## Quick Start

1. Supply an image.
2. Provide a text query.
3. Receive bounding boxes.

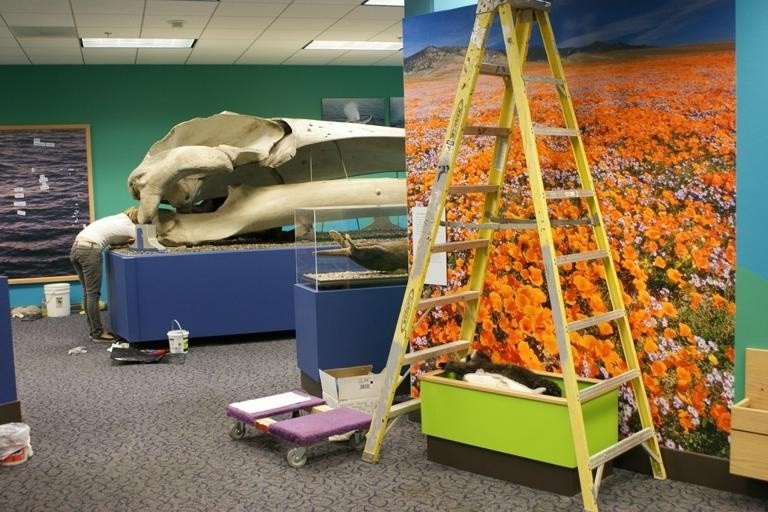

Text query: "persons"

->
[71,206,140,344]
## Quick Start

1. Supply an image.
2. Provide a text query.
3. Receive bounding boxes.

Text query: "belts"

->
[74,240,101,249]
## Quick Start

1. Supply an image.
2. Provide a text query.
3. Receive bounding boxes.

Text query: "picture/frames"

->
[389,96,404,127]
[320,97,386,127]
[0,124,95,285]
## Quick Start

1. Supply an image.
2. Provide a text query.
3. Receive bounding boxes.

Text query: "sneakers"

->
[93,333,120,342]
[89,333,93,339]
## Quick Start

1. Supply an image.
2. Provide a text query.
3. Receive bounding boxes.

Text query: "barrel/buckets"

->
[44,283,71,318]
[167,318,190,354]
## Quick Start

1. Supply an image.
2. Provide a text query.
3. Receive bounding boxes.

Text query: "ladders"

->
[361,1,667,512]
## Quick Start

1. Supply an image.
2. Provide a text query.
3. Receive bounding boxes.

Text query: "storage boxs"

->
[417,363,617,497]
[318,365,404,417]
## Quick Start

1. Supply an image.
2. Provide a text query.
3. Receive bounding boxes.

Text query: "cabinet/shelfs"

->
[291,204,411,399]
[105,229,407,343]
